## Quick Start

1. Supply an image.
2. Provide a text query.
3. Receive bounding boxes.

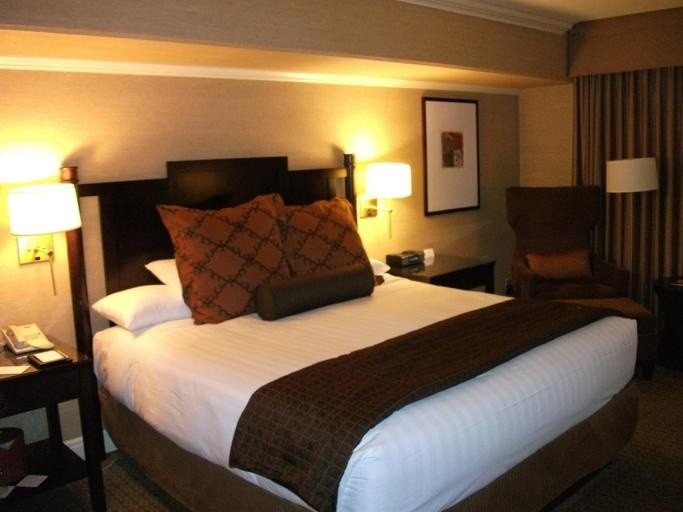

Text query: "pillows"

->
[275,196,375,281]
[253,262,374,320]
[142,258,182,290]
[367,255,392,278]
[155,192,292,325]
[524,249,594,282]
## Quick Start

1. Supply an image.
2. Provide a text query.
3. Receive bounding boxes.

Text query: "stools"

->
[542,295,656,381]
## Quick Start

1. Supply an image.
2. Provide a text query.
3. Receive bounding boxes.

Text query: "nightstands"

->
[0,333,108,511]
[386,252,497,294]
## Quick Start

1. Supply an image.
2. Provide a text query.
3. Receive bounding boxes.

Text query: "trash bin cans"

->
[0,427,30,485]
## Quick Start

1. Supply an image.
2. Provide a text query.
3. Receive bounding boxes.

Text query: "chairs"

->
[505,185,629,300]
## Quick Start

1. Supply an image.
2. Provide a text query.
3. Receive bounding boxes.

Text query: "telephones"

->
[1,323,54,355]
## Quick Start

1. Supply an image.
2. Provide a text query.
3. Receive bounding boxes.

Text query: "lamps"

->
[6,182,83,296]
[87,284,194,332]
[358,161,412,240]
[605,156,660,194]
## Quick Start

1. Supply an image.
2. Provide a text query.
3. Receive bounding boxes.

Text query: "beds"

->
[60,154,640,512]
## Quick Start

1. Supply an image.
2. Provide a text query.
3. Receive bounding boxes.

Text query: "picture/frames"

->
[421,96,481,216]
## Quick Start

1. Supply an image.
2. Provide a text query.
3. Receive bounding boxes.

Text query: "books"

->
[28,349,73,369]
